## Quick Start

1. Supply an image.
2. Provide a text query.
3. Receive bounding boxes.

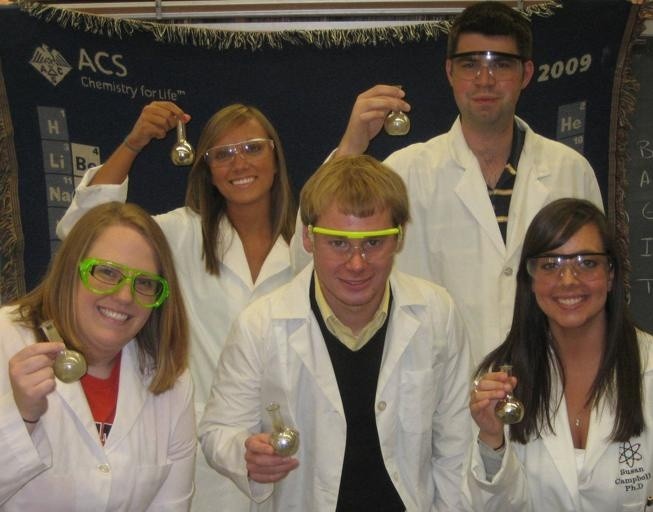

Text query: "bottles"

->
[171,117,195,167]
[38,319,86,385]
[383,84,411,136]
[265,403,299,455]
[494,363,526,426]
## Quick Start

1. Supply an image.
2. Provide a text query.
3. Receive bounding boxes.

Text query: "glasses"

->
[451,50,526,80]
[79,257,171,309]
[308,224,404,257]
[205,138,275,167]
[527,251,617,284]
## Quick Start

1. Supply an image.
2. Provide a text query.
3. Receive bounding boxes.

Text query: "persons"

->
[55,103,290,422]
[466,197,653,511]
[197,154,476,512]
[0,200,197,512]
[290,6,607,378]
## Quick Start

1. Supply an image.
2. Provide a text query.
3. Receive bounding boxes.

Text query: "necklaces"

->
[547,328,603,431]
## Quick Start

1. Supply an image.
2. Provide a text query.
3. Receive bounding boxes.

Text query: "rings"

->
[472,377,482,391]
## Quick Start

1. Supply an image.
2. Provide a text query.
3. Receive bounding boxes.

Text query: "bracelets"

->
[22,414,42,426]
[122,135,145,155]
